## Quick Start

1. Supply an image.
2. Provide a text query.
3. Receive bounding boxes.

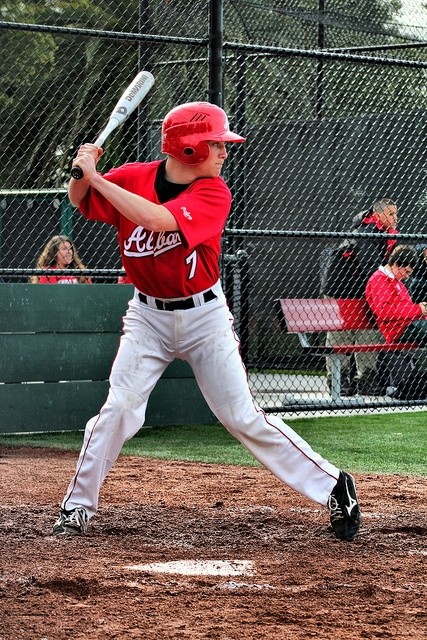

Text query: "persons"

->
[32,236,93,284]
[318,198,399,396]
[365,245,427,347]
[52,100,360,542]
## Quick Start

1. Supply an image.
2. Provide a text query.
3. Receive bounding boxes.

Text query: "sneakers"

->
[326,369,355,396]
[328,471,360,540]
[356,368,380,396]
[49,507,88,538]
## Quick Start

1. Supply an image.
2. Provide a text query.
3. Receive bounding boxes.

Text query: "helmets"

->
[161,101,246,166]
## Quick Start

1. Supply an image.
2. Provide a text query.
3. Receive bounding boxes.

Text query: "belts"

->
[137,289,217,311]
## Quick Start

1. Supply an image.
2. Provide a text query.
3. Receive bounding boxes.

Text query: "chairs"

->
[275,297,427,407]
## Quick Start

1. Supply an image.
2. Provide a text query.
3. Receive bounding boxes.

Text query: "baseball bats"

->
[70,70,155,180]
